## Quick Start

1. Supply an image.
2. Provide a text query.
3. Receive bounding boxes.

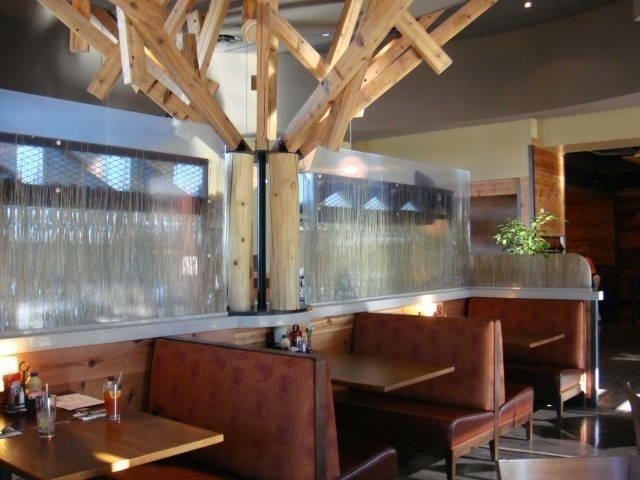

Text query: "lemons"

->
[109,390,122,399]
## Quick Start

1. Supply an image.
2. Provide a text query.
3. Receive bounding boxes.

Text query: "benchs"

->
[469,298,589,420]
[314,356,398,479]
[498,384,534,451]
[350,313,499,479]
[117,339,326,480]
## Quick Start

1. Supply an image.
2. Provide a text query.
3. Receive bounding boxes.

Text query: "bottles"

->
[107,376,120,392]
[2,373,27,403]
[433,303,449,318]
[17,359,30,384]
[9,380,27,417]
[417,311,423,316]
[271,325,314,353]
[299,266,309,310]
[24,370,44,397]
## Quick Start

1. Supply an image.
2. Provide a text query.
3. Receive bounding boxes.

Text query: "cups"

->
[103,382,123,422]
[32,394,57,439]
[37,433,58,447]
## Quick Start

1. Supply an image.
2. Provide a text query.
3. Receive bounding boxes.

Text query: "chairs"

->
[624,380,639,451]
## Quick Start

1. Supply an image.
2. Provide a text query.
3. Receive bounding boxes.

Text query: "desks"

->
[296,350,455,479]
[0,411,226,479]
[494,454,640,480]
[499,333,568,421]
